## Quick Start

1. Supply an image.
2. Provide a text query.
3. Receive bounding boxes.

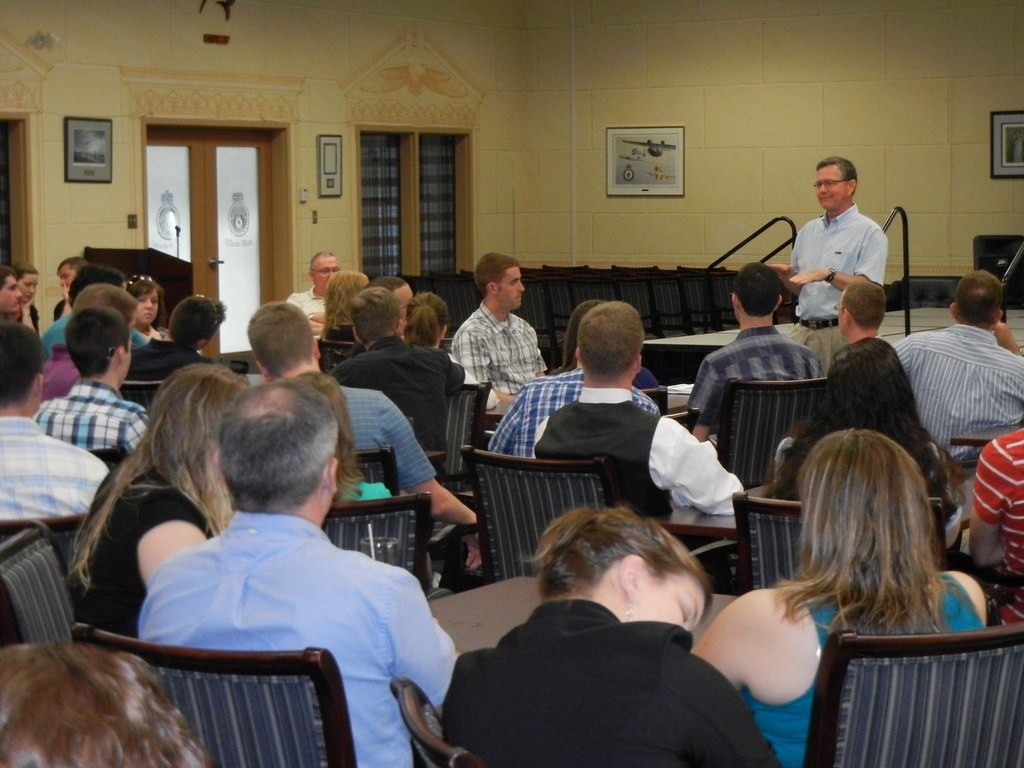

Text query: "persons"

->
[488,299,661,459]
[247,301,482,571]
[690,429,986,768]
[286,251,341,336]
[451,253,549,401]
[328,286,465,452]
[0,260,40,332]
[532,301,744,519]
[33,305,149,454]
[767,157,888,378]
[78,366,251,639]
[319,270,413,343]
[0,318,110,521]
[439,507,783,768]
[766,337,958,518]
[125,295,225,382]
[834,281,886,345]
[40,256,165,401]
[0,642,210,768]
[966,428,1024,626]
[404,291,497,409]
[139,380,456,768]
[890,269,1024,461]
[686,261,823,443]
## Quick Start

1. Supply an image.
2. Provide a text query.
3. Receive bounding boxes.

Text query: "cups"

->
[360,537,399,569]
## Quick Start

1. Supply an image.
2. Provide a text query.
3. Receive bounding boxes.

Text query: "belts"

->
[798,318,838,330]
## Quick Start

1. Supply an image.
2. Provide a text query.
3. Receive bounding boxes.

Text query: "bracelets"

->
[825,268,835,283]
[309,313,313,319]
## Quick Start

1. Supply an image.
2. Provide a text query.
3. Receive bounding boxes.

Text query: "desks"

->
[641,306,1024,394]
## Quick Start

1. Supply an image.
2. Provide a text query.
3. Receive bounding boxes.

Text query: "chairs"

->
[0,233,1024,768]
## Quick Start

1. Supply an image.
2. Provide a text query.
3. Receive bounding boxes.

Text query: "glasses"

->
[126,273,154,292]
[813,179,847,189]
[309,266,341,274]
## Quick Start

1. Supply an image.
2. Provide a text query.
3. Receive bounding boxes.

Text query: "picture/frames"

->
[315,134,342,198]
[64,117,113,183]
[989,110,1024,178]
[606,126,685,198]
